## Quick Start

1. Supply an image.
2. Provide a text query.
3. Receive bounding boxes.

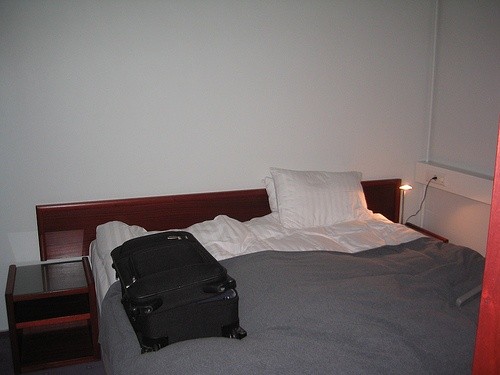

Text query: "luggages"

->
[111,231,248,353]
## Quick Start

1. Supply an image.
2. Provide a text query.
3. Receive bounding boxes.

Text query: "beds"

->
[36,178,486,375]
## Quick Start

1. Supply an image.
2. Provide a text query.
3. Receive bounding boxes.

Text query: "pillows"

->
[263,166,371,230]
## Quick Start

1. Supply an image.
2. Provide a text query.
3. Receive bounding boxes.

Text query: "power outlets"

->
[424,171,449,188]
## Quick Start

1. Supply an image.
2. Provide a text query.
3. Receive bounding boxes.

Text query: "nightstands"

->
[4,257,99,375]
